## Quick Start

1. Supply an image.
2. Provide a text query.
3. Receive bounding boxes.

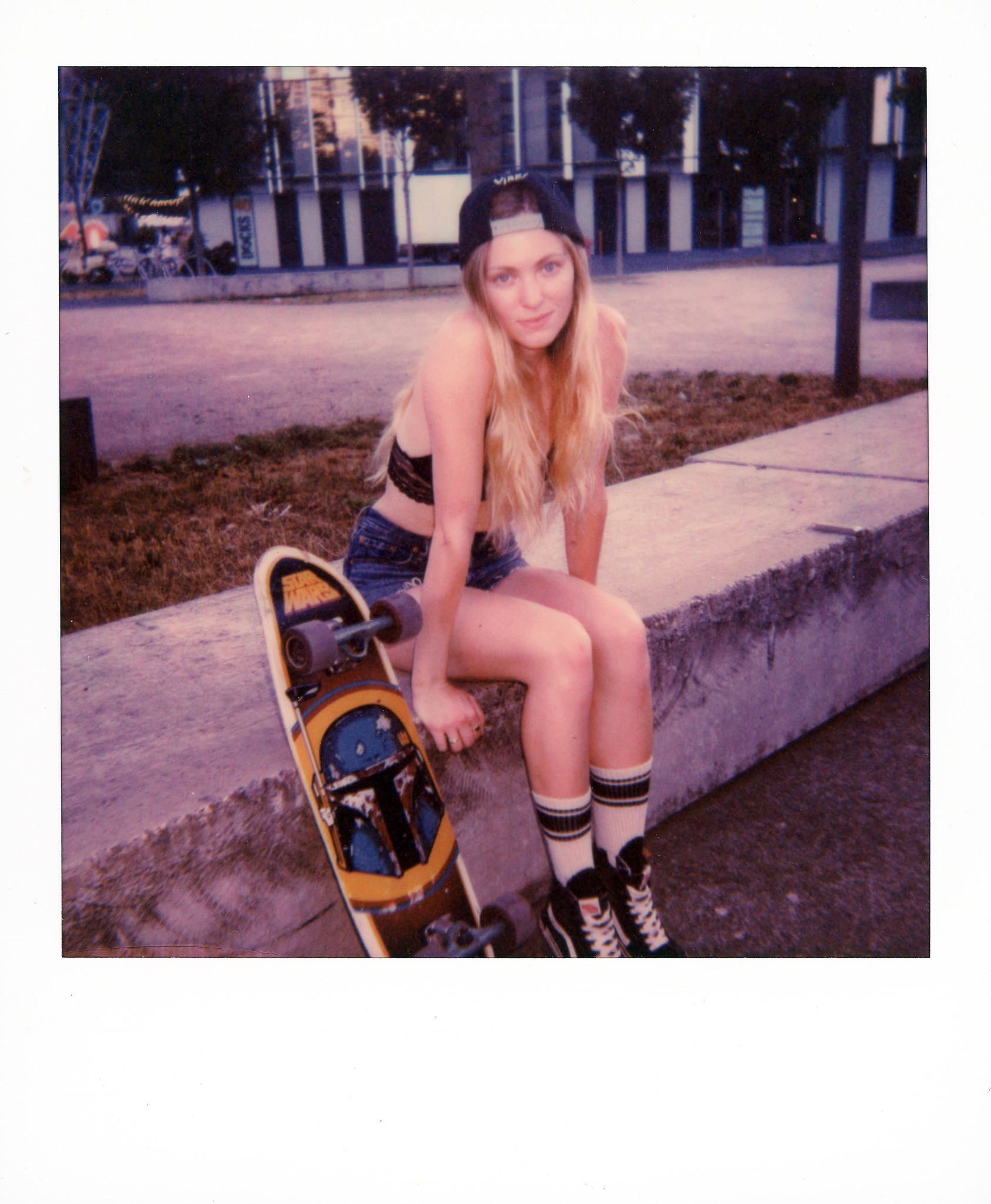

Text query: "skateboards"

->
[251,544,537,957]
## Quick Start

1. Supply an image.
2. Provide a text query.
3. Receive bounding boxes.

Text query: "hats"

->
[455,166,587,272]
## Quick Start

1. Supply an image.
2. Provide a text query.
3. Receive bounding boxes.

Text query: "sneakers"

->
[597,864,688,958]
[538,886,637,959]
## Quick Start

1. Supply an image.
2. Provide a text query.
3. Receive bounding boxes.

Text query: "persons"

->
[342,169,692,958]
[135,223,190,260]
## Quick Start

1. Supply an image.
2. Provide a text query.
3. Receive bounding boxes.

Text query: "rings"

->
[449,737,461,744]
[472,724,481,732]
